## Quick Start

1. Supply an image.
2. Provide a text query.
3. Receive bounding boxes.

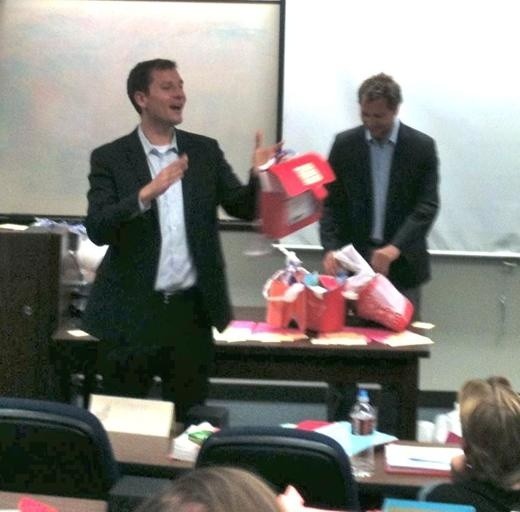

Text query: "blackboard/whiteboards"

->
[0,0,285,233]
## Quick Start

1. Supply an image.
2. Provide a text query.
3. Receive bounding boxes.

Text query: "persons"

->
[136,462,305,512]
[419,375,520,511]
[77,58,286,425]
[316,70,442,440]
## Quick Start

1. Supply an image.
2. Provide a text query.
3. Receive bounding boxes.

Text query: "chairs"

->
[0,395,520,512]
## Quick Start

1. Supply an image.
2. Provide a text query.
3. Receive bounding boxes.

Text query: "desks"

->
[48,303,431,441]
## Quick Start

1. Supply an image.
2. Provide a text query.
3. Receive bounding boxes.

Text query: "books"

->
[169,419,222,462]
[385,443,468,477]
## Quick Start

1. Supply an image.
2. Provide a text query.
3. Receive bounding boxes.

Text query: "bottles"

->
[350,388,376,479]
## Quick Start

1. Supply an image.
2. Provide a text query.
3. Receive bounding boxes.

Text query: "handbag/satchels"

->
[262,266,415,333]
[258,148,337,236]
